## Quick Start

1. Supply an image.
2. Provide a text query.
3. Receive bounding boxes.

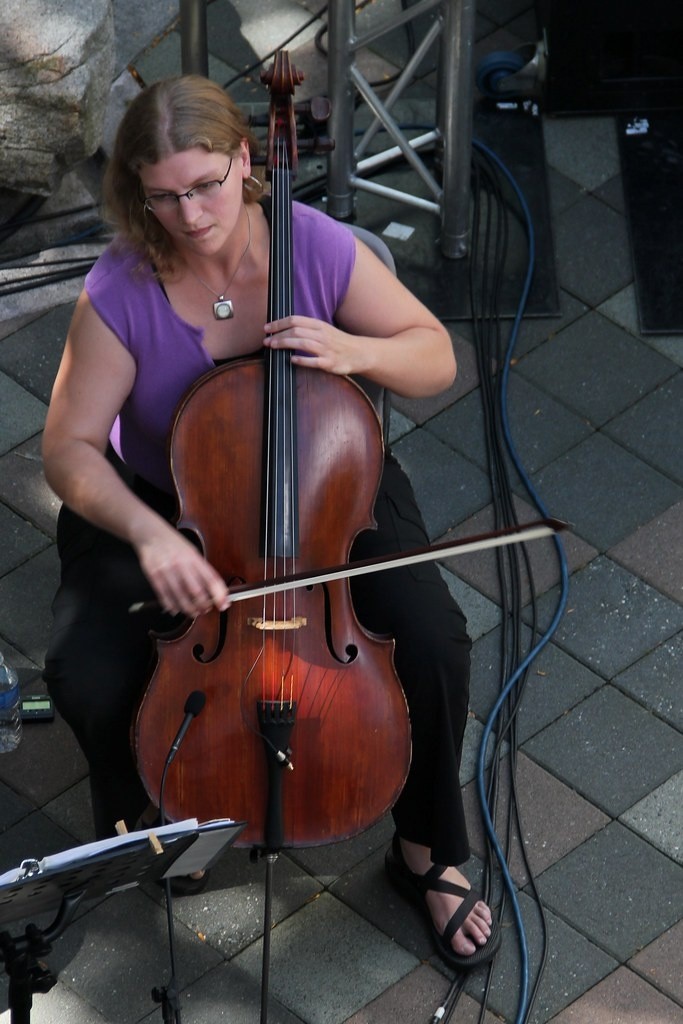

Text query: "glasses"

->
[143,158,233,210]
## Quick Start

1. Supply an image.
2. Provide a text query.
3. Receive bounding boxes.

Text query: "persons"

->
[42,73,503,966]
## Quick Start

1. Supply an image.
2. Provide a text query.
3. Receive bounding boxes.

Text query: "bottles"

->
[0,652,23,754]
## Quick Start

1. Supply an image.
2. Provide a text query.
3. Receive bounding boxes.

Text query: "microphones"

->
[167,692,206,768]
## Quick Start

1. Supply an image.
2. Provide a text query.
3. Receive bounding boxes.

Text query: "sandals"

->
[142,805,209,895]
[383,825,504,969]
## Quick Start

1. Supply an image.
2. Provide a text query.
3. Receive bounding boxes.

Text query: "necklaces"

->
[172,203,252,321]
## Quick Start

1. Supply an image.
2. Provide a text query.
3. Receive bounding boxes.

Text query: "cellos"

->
[133,50,411,1024]
